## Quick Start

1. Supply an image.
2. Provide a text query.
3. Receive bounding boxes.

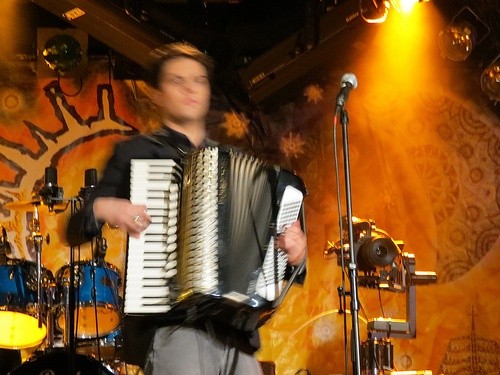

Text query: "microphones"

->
[38,167,59,206]
[335,73,358,111]
[79,168,97,200]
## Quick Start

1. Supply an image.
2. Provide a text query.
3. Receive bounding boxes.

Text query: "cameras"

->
[335,216,404,272]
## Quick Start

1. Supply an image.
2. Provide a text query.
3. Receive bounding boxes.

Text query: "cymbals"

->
[4,199,67,212]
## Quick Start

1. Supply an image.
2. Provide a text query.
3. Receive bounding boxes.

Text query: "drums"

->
[11,350,113,375]
[0,260,54,350]
[56,260,122,338]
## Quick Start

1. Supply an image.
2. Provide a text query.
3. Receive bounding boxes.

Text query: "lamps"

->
[36,27,88,79]
[480,54,500,106]
[438,6,491,61]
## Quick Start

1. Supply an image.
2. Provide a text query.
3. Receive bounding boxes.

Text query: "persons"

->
[64,45,306,374]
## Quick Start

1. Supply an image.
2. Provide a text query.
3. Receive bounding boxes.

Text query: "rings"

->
[133,215,141,222]
